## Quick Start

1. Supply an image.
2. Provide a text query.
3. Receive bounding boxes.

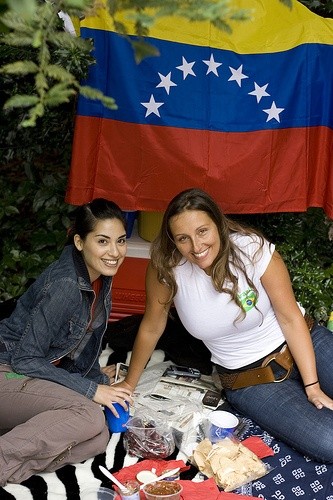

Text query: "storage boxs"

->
[91,219,154,323]
[171,407,213,444]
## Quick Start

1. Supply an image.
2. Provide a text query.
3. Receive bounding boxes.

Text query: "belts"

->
[219,312,314,391]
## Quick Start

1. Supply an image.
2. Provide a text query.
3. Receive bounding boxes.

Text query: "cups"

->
[117,480,141,500]
[78,486,121,500]
[104,400,129,432]
[207,410,239,444]
[143,480,183,500]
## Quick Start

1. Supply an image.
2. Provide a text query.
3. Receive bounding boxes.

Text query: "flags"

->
[59,0,333,218]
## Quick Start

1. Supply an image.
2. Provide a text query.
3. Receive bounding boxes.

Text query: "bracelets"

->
[303,380,319,388]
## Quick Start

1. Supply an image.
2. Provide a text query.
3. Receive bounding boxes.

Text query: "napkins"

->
[241,436,275,458]
[113,459,265,500]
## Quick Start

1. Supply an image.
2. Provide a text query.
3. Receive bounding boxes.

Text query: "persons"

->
[0,199,135,488]
[113,188,333,464]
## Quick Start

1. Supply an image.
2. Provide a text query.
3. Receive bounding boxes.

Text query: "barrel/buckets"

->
[122,210,137,239]
[140,210,166,242]
